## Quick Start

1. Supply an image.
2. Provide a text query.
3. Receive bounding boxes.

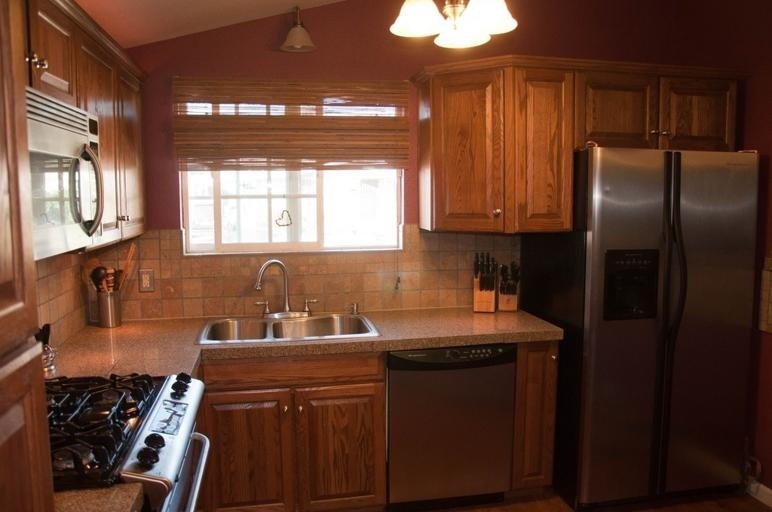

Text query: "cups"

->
[95,289,124,328]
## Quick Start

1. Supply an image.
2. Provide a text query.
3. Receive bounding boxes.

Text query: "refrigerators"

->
[521,146,758,512]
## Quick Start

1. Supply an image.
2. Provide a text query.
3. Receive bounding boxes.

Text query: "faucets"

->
[254,258,291,311]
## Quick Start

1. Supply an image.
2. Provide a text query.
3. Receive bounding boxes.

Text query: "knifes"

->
[498,261,519,296]
[473,252,496,292]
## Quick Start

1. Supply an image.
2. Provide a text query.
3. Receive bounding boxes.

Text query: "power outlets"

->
[137,269,155,292]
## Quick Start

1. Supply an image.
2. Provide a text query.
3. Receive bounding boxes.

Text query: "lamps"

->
[388,0,519,50]
[280,6,317,54]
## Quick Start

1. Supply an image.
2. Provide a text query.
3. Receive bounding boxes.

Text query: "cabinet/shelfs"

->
[21,0,79,108]
[411,56,578,235]
[200,379,388,512]
[66,24,146,255]
[2,0,56,512]
[574,57,741,152]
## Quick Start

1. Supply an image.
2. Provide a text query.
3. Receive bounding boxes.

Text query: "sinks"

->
[271,314,381,341]
[194,317,270,344]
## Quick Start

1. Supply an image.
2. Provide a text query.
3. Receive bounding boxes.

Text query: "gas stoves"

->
[43,366,207,491]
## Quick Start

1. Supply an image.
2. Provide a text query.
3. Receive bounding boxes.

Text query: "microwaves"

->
[23,84,104,263]
